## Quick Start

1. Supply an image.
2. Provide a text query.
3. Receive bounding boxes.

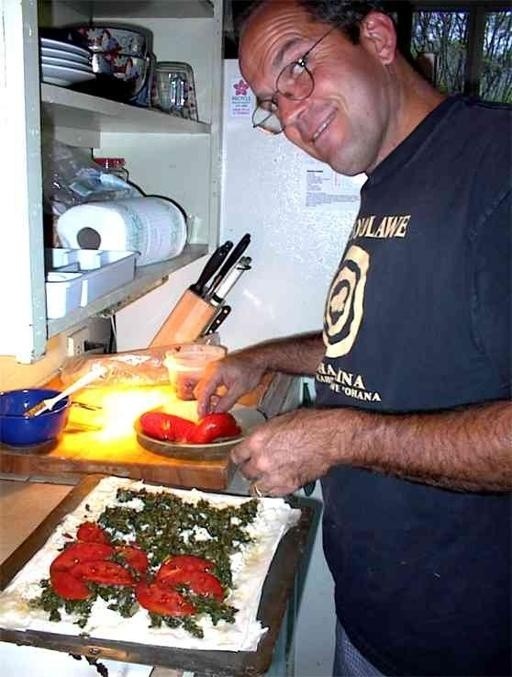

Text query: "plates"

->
[39,36,97,89]
[133,398,268,448]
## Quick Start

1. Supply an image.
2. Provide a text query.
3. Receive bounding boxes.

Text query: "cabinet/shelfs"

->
[0,0,223,363]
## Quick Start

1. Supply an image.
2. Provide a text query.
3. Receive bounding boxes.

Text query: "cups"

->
[152,61,200,120]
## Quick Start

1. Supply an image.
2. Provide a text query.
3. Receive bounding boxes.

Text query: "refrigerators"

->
[221,56,369,347]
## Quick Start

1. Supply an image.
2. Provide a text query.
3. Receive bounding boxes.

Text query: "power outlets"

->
[67,326,88,356]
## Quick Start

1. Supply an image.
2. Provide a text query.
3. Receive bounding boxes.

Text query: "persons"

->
[194,1,511,677]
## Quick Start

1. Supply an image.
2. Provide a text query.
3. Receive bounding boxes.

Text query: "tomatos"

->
[46,520,222,618]
[136,408,239,442]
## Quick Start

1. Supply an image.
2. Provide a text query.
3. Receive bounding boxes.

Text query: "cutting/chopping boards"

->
[0,471,324,672]
[3,340,296,491]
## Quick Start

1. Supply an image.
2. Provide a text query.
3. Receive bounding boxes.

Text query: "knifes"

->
[194,232,259,339]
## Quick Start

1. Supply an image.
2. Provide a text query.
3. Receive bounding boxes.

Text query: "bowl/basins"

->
[63,23,152,103]
[0,388,71,451]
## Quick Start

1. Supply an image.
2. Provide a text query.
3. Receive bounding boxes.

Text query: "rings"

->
[252,481,263,498]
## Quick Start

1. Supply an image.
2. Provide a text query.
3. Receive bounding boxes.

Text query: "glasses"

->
[252,22,338,134]
